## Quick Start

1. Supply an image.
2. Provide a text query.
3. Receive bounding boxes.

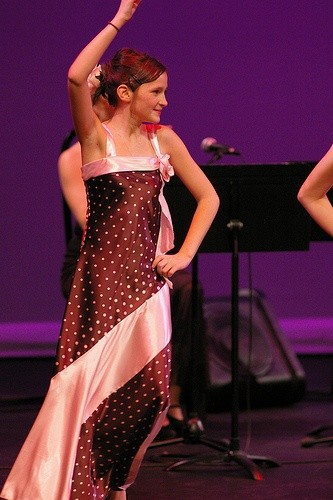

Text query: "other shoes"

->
[166,404,186,432]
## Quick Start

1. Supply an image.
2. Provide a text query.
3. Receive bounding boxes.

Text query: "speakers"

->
[202,286,306,401]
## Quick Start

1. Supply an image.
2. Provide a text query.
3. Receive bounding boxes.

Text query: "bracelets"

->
[107,20,121,33]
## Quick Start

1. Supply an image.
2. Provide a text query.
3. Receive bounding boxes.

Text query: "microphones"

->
[200,136,243,156]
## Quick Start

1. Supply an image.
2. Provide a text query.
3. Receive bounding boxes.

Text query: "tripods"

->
[165,219,283,481]
[142,252,227,455]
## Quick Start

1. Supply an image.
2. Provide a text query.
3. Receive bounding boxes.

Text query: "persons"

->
[2,0,221,499]
[296,143,333,238]
[55,63,184,431]
